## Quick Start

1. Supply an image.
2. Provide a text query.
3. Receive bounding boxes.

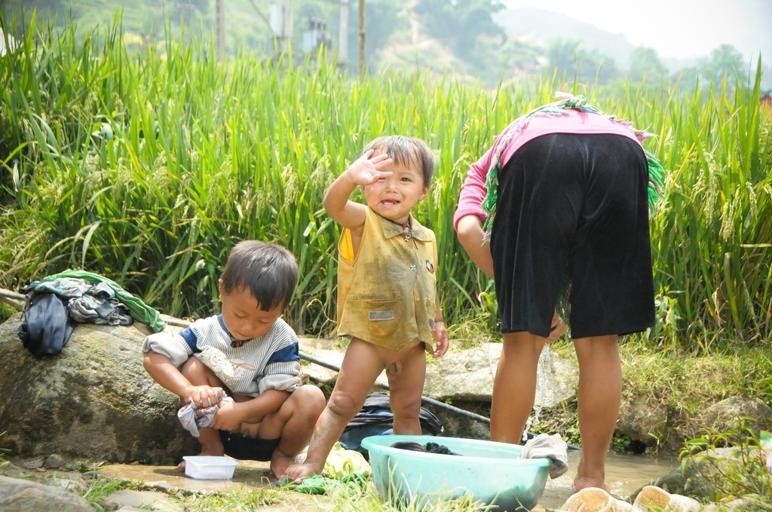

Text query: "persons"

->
[453,93,667,492]
[281,135,449,484]
[142,240,326,480]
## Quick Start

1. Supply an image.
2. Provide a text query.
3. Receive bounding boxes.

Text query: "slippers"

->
[632,483,701,512]
[545,485,632,512]
